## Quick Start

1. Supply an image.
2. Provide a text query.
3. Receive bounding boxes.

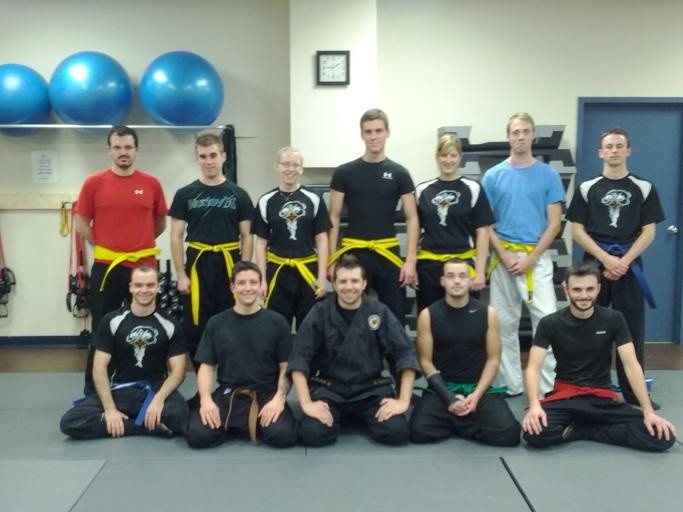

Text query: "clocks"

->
[315,50,350,85]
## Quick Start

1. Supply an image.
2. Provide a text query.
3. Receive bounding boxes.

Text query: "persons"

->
[181,259,302,449]
[253,146,332,332]
[568,127,668,410]
[71,124,167,399]
[521,262,679,452]
[168,134,255,373]
[480,111,566,402]
[327,108,421,330]
[413,133,497,316]
[59,264,190,438]
[408,258,520,448]
[286,254,421,446]
